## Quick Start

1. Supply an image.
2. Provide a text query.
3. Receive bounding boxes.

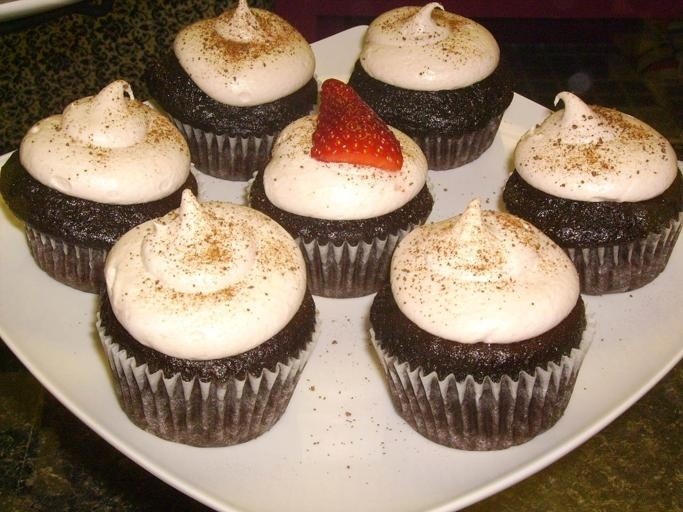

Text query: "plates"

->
[0,20,683,512]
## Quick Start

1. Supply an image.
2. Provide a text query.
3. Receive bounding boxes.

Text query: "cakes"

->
[503,91,682,295]
[143,1,319,181]
[370,196,586,452]
[0,78,197,295]
[100,187,316,448]
[348,3,514,170]
[249,78,434,299]
